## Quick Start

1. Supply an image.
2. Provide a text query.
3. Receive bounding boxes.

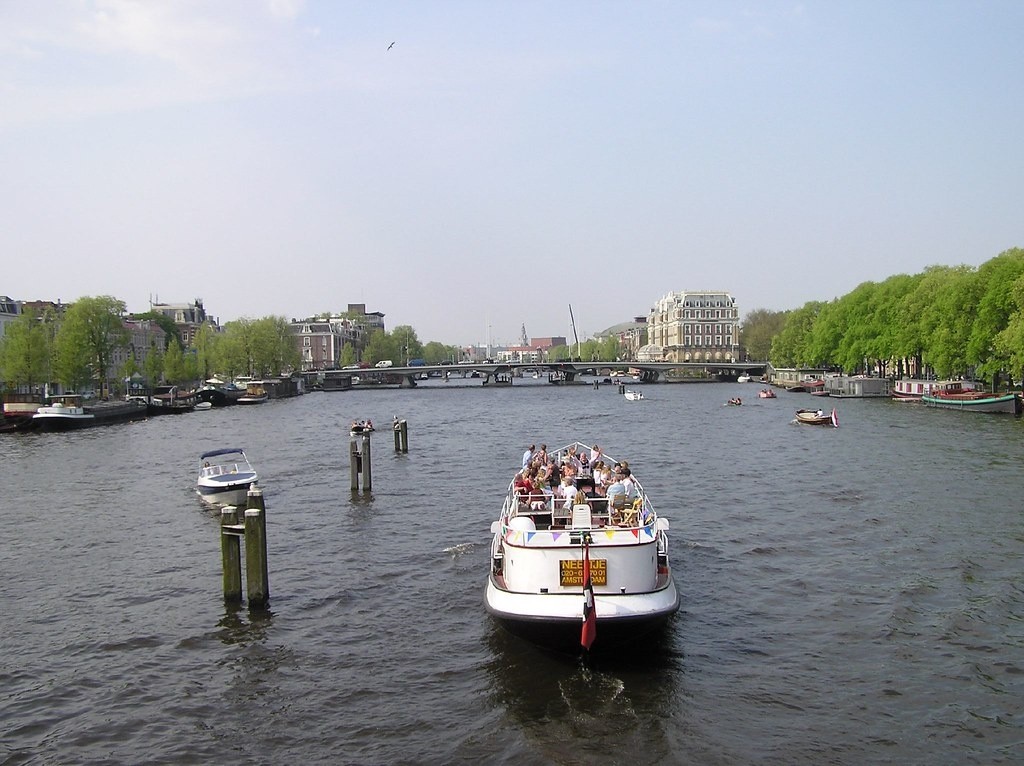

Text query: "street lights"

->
[400,344,407,362]
[489,324,492,359]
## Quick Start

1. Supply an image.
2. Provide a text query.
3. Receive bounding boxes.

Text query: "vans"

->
[374,360,393,368]
[409,359,425,367]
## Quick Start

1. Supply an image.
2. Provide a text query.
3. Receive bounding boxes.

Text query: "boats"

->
[351,376,360,385]
[483,445,683,630]
[795,408,839,428]
[923,383,1022,416]
[350,423,373,434]
[193,401,212,411]
[31,395,95,432]
[402,370,642,384]
[758,389,777,398]
[891,378,972,403]
[193,447,260,507]
[736,371,751,383]
[236,388,268,404]
[785,385,805,392]
[724,403,745,406]
[811,390,831,397]
[625,389,643,401]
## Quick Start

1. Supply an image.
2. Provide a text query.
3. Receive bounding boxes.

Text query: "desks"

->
[575,474,596,497]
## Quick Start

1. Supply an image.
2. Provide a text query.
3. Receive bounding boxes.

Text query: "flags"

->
[832,408,839,428]
[581,542,597,650]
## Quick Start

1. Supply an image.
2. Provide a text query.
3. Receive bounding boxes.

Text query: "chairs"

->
[610,495,626,524]
[620,498,642,528]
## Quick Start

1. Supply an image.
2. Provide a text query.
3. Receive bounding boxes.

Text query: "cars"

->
[82,391,96,399]
[505,359,522,363]
[343,364,361,369]
[126,395,163,406]
[483,358,499,364]
[437,359,453,366]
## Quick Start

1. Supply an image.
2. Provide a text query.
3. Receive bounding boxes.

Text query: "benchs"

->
[517,494,611,525]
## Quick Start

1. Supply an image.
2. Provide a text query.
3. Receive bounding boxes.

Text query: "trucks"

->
[93,389,109,400]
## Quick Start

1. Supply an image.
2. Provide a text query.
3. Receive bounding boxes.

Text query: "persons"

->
[759,388,776,398]
[513,443,640,528]
[728,397,742,406]
[350,418,374,433]
[816,409,823,419]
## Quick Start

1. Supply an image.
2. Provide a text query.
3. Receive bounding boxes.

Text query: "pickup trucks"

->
[457,359,474,365]
